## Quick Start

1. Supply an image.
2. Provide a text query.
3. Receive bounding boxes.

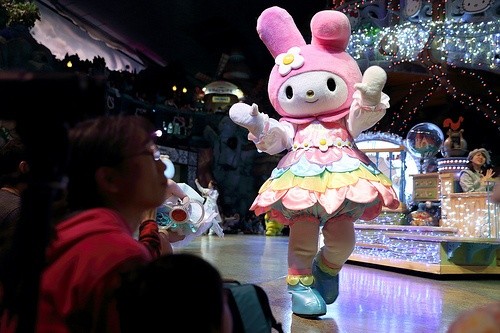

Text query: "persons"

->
[0,122,47,228]
[120,252,233,333]
[458,148,495,192]
[194,177,226,238]
[150,176,191,258]
[0,114,170,333]
[219,208,291,236]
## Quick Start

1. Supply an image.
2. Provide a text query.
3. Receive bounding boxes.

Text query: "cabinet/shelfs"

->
[413,173,439,200]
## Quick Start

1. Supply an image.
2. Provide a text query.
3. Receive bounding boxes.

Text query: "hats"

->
[468,148,491,164]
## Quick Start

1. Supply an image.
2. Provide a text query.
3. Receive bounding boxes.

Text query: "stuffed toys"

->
[229,7,390,315]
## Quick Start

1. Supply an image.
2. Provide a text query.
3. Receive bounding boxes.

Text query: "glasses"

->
[130,141,160,161]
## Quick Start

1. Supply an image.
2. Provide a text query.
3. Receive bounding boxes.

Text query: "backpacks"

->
[222,279,284,333]
